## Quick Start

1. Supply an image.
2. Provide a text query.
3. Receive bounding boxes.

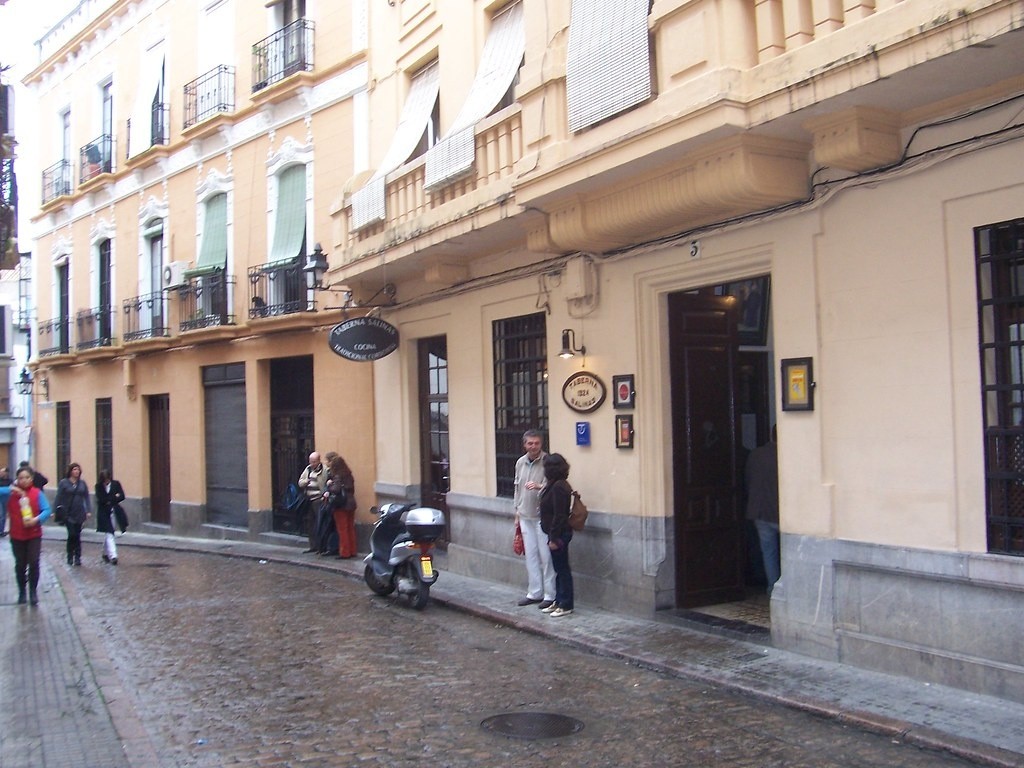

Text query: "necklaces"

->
[71,478,78,488]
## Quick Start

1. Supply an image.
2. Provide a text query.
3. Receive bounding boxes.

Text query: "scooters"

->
[363,501,447,611]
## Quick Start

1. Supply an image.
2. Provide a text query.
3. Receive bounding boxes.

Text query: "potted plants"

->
[85,144,102,177]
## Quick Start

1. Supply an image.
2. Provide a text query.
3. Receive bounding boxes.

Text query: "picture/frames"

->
[782,357,816,412]
[616,414,633,448]
[722,277,771,346]
[612,374,636,409]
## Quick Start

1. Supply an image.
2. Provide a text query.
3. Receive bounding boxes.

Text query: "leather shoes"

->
[516,595,543,605]
[539,600,554,608]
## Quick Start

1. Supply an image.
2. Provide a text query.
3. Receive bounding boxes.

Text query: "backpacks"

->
[285,482,310,515]
[561,480,589,530]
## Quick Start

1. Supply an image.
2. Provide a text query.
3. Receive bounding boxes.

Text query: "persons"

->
[746,423,780,597]
[0,466,52,605]
[50,463,92,565]
[95,469,129,565]
[0,461,49,537]
[298,451,357,560]
[539,453,574,616]
[513,429,556,608]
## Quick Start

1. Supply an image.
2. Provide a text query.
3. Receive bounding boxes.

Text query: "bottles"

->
[19,493,34,525]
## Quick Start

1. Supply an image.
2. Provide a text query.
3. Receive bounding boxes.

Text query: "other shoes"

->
[18,592,26,603]
[30,592,38,605]
[112,558,117,565]
[75,557,81,565]
[67,557,73,564]
[321,550,335,557]
[102,554,110,562]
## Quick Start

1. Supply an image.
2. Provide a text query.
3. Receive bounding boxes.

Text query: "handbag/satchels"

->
[54,505,68,522]
[328,485,348,507]
[512,521,525,555]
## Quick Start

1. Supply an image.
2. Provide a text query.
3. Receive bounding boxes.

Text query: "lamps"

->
[14,368,48,401]
[557,329,586,359]
[304,243,351,292]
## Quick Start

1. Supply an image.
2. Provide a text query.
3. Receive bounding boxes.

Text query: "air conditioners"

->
[165,261,189,288]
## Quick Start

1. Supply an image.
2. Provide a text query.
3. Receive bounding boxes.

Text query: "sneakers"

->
[542,601,559,612]
[550,607,571,616]
